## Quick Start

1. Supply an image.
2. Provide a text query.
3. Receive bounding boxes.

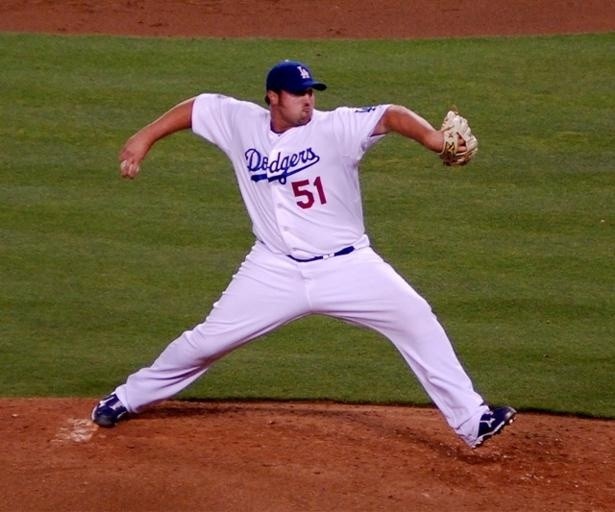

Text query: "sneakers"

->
[473,406,516,449]
[91,392,127,427]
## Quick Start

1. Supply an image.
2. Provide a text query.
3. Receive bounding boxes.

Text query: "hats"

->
[266,59,327,93]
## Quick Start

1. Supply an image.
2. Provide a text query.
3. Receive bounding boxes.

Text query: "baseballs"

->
[120,160,140,177]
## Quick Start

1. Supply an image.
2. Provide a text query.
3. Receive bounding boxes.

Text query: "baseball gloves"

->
[437,110,479,166]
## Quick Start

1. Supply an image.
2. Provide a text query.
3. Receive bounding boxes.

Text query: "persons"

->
[92,60,519,450]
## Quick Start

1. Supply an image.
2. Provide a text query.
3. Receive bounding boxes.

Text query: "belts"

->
[289,246,354,263]
